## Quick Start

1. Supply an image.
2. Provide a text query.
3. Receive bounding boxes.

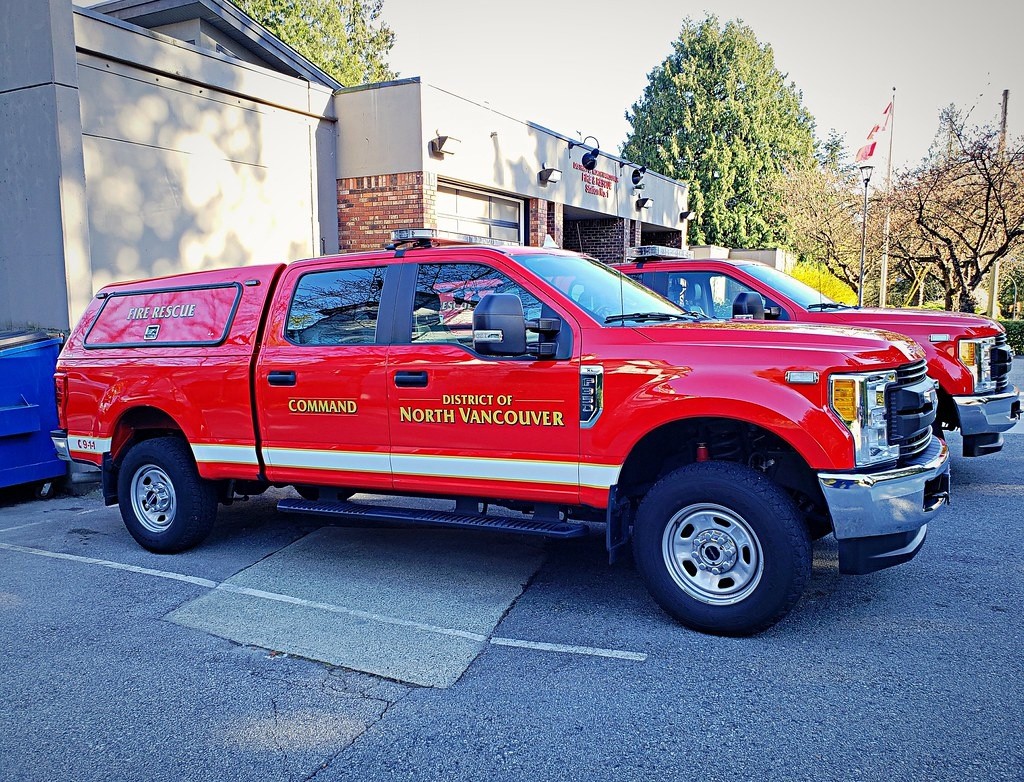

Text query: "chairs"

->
[296,296,377,344]
[415,322,462,346]
[682,283,706,316]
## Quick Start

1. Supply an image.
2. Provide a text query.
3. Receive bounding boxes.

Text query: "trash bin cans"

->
[0,330,68,500]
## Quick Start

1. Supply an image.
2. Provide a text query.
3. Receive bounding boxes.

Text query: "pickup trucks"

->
[433,242,1024,458]
[50,227,950,639]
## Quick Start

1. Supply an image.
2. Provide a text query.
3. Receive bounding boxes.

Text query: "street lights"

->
[858,164,875,308]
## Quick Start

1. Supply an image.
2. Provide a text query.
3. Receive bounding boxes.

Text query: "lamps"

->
[638,197,654,210]
[680,211,696,221]
[569,135,600,170]
[633,184,645,192]
[540,168,563,184]
[438,135,462,155]
[620,155,647,185]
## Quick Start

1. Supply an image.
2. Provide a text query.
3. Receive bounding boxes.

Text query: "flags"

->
[854,102,892,164]
